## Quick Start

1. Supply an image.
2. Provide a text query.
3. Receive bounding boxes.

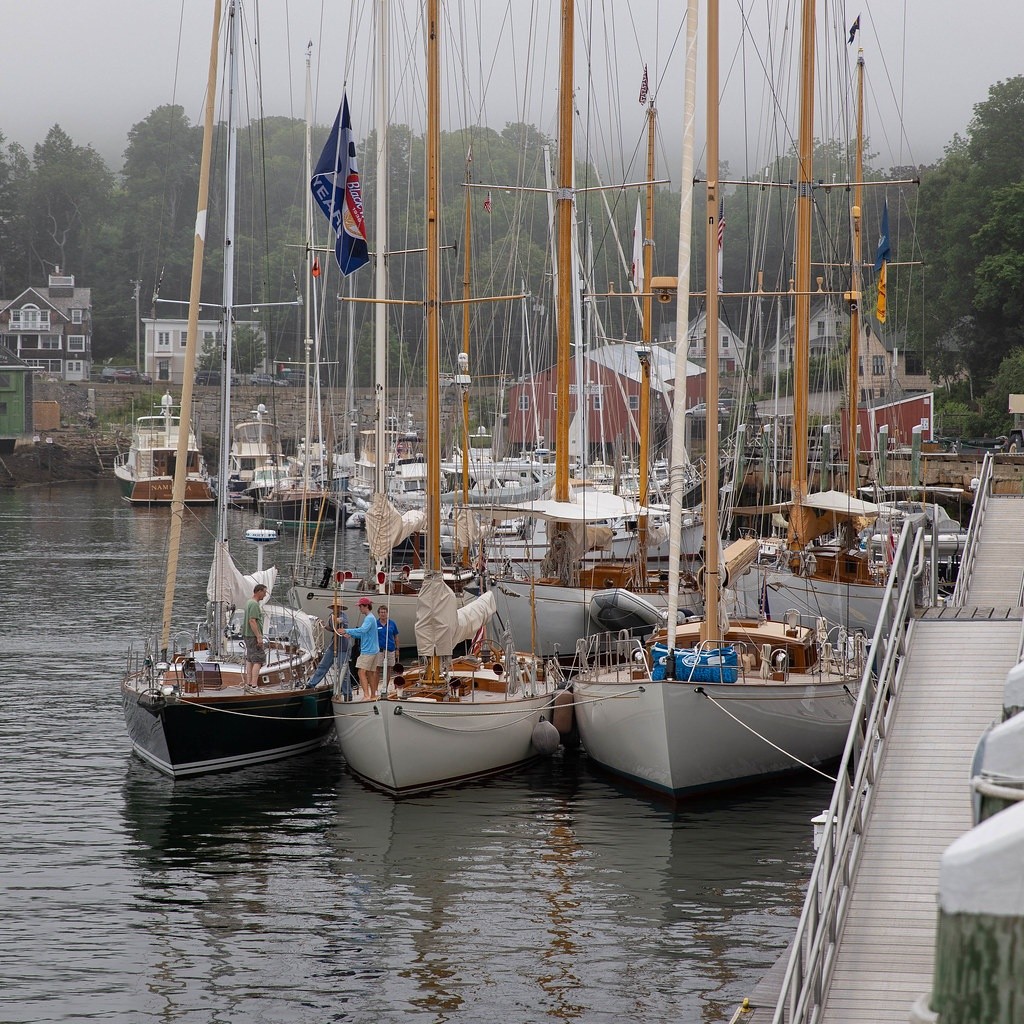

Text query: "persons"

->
[301,597,399,702]
[242,584,267,694]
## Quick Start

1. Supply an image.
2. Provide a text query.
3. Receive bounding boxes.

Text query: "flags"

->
[873,192,891,275]
[717,196,726,253]
[876,258,886,324]
[309,89,370,277]
[312,252,320,278]
[639,63,650,106]
[886,512,894,567]
[846,14,860,44]
[632,196,644,293]
[484,190,491,213]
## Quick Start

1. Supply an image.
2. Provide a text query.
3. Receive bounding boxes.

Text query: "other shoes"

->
[344,694,352,701]
[303,685,311,689]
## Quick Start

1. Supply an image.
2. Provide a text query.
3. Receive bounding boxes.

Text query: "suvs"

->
[194,372,241,385]
[438,374,454,386]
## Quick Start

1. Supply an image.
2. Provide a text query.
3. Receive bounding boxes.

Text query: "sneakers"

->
[246,684,252,691]
[250,685,264,694]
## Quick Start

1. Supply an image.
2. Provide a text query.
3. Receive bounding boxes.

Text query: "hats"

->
[326,599,348,610]
[355,597,373,606]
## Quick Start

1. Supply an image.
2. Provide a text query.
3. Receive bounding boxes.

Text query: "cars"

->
[274,368,325,387]
[115,367,153,385]
[250,373,291,386]
[685,403,730,418]
[718,399,746,414]
[100,365,117,383]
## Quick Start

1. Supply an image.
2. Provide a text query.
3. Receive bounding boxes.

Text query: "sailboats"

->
[114,0,968,799]
